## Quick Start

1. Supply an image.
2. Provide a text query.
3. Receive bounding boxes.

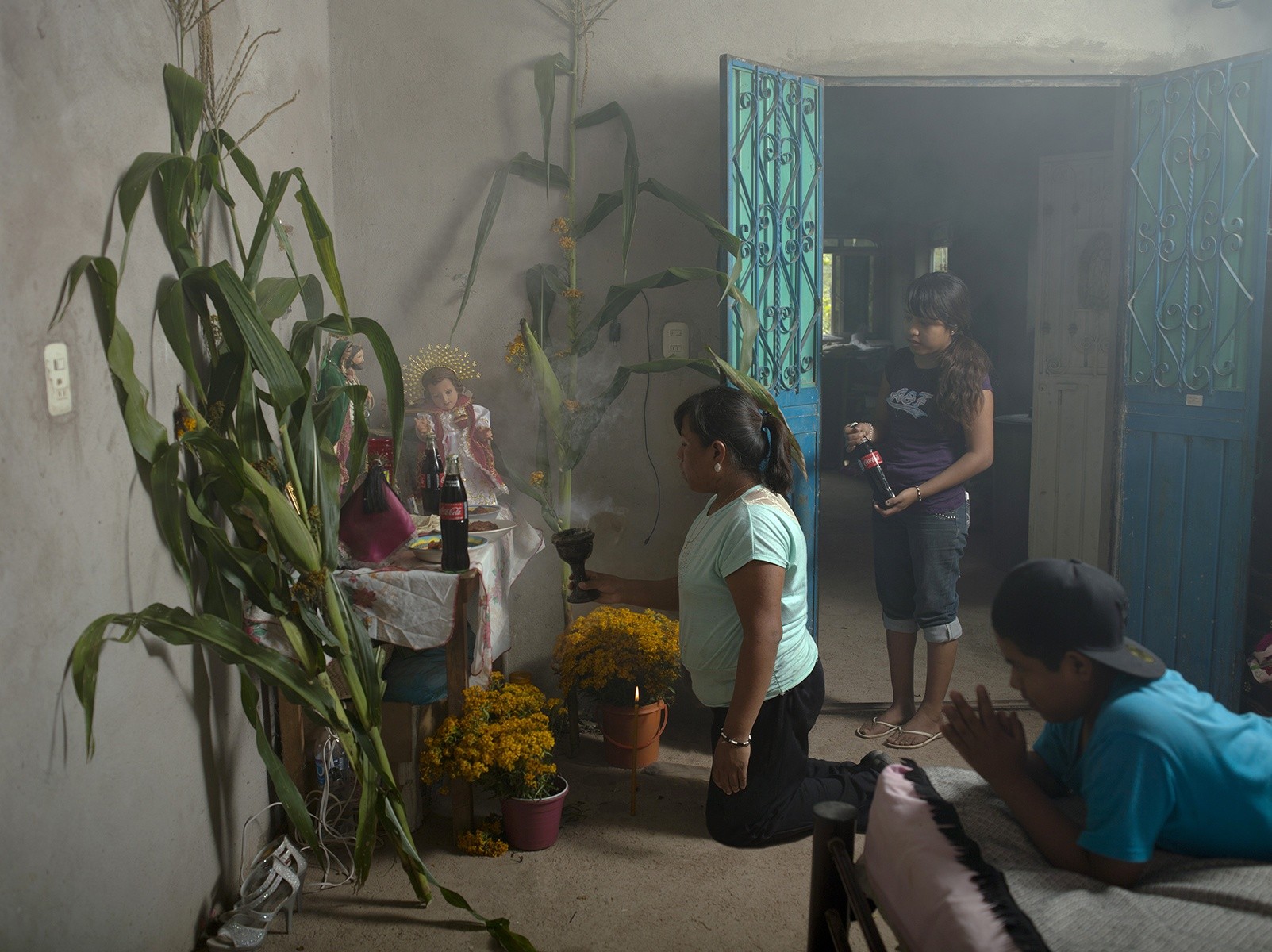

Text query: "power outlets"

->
[662,321,689,360]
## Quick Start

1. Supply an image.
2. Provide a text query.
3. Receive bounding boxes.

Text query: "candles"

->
[629,687,640,816]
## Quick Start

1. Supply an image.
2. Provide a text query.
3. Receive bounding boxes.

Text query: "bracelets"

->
[720,728,751,746]
[864,422,873,441]
[916,485,922,501]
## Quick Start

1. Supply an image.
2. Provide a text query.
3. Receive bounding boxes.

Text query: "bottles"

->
[849,421,897,511]
[421,433,445,515]
[439,455,471,573]
[314,728,356,843]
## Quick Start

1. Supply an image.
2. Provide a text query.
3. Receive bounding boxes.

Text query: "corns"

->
[520,316,566,434]
[175,381,319,578]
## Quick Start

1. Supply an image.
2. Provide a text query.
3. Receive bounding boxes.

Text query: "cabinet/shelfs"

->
[820,345,892,468]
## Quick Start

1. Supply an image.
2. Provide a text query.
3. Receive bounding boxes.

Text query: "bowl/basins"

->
[405,534,488,563]
[466,519,518,543]
[468,505,501,518]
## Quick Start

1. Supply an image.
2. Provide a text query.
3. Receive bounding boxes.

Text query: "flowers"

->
[417,671,568,859]
[552,601,687,744]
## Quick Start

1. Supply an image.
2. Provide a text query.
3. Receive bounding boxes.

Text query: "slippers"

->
[885,726,944,749]
[856,718,906,738]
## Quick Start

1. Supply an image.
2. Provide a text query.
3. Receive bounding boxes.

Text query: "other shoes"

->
[860,749,895,770]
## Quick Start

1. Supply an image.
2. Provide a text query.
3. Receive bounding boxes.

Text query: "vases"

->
[602,704,668,768]
[500,776,568,851]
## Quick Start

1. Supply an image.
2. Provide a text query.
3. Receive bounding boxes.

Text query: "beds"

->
[805,756,1272,950]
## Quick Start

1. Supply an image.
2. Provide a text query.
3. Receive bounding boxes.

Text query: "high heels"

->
[219,835,306,923]
[205,854,300,950]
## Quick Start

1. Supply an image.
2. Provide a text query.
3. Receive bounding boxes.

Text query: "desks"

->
[276,506,543,796]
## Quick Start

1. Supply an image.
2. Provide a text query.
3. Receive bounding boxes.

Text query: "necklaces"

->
[683,482,755,550]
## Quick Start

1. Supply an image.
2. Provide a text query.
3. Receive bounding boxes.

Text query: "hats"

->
[993,560,1166,678]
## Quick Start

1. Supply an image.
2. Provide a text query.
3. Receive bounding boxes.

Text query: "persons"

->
[840,271,994,749]
[939,558,1272,889]
[674,387,887,849]
[346,345,372,428]
[331,339,351,495]
[415,368,509,506]
